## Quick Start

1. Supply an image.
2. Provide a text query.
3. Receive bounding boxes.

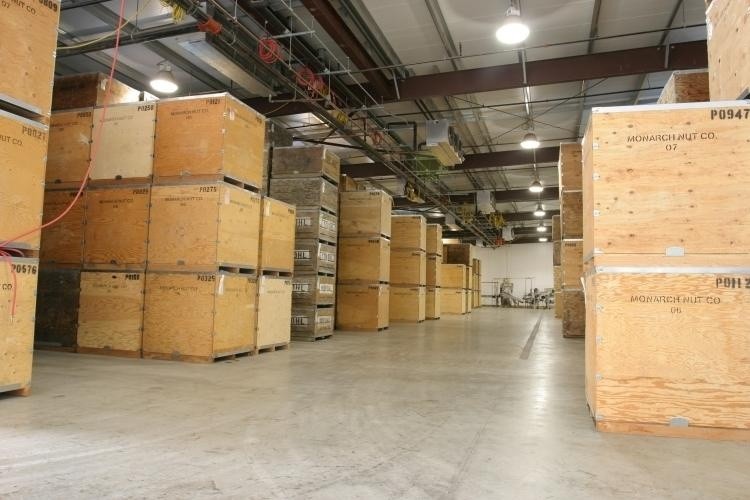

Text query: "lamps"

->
[495,1,548,242]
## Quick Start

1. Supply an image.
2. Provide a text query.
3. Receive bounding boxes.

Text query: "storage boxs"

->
[551,0,749,441]
